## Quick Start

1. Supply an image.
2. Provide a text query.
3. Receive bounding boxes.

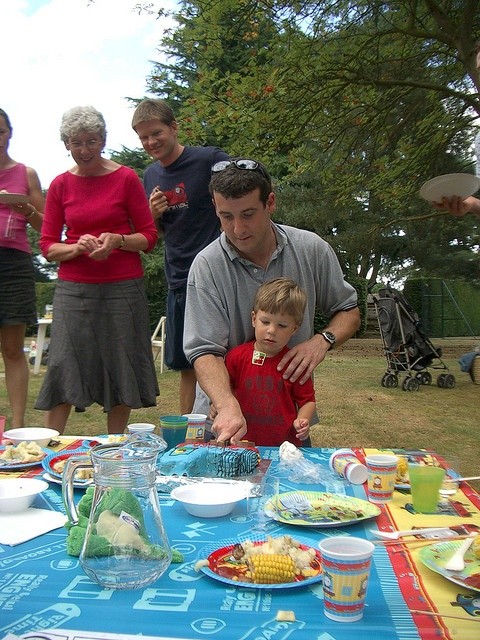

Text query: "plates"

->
[42,449,94,482]
[264,491,381,527]
[0,445,54,468]
[394,464,462,489]
[419,540,480,592]
[83,434,131,449]
[196,533,322,589]
[0,193,31,205]
[42,472,88,489]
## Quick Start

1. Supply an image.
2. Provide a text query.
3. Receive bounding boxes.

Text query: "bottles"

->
[62,443,173,589]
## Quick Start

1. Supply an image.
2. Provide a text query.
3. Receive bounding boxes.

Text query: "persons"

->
[209,276,315,447]
[34,105,159,436]
[431,43,480,220]
[0,108,45,430]
[182,157,362,447]
[132,99,229,418]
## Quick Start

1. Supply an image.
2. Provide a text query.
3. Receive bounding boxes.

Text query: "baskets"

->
[469,355,480,386]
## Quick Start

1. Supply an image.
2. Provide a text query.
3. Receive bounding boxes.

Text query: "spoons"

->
[369,527,449,540]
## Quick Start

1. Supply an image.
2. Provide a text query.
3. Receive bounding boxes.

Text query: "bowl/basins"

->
[0,478,49,512]
[171,484,248,517]
[3,428,59,448]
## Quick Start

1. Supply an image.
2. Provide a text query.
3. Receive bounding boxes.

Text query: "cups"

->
[319,536,375,623]
[159,415,188,452]
[128,423,155,435]
[409,466,445,512]
[329,449,367,484]
[365,455,398,505]
[0,415,6,438]
[183,414,207,442]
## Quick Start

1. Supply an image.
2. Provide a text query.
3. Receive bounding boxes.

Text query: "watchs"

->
[317,330,336,351]
[116,233,125,250]
[25,210,37,220]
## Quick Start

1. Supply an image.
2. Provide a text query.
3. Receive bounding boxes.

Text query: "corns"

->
[247,552,297,583]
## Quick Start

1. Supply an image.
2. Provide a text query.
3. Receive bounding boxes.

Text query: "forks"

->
[445,532,478,571]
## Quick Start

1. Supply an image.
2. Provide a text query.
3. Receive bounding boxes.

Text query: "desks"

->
[35,317,54,376]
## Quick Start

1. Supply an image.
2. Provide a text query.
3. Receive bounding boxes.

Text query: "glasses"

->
[66,141,102,148]
[211,159,266,178]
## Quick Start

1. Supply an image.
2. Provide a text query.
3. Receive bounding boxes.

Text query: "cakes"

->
[156,439,262,480]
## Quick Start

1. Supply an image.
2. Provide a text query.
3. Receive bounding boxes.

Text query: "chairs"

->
[149,317,176,374]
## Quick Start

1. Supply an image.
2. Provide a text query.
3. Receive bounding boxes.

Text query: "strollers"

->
[371,288,455,392]
